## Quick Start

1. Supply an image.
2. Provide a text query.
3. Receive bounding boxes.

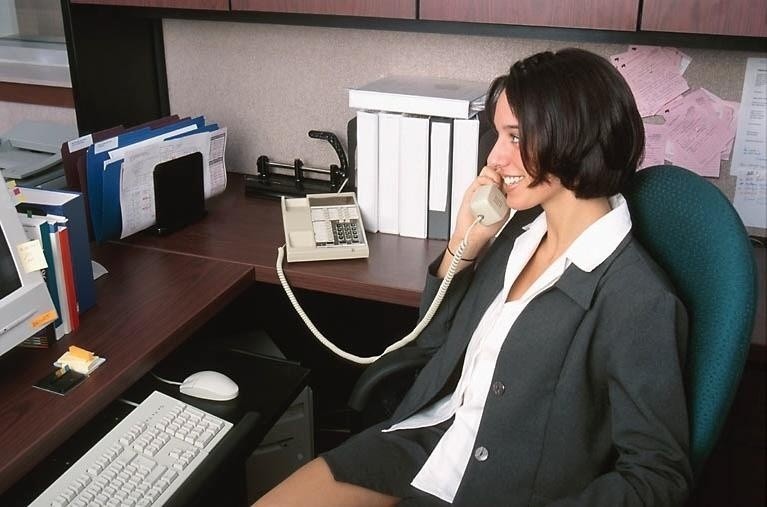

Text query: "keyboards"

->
[27,389,234,506]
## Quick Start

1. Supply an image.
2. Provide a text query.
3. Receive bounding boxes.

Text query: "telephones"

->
[469,185,508,226]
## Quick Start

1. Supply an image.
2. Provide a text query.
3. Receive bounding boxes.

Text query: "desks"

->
[108,170,767,364]
[0,247,256,494]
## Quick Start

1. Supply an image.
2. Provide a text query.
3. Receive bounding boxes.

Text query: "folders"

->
[348,75,490,241]
[61,114,227,246]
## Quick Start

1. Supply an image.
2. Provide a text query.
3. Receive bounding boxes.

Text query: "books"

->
[14,188,95,353]
[350,77,492,241]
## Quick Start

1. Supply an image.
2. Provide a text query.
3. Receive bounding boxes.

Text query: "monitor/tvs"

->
[0,168,59,355]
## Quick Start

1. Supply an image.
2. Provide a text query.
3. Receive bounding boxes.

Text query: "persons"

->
[250,47,690,507]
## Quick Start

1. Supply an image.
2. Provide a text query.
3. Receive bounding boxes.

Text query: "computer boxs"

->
[239,333,315,505]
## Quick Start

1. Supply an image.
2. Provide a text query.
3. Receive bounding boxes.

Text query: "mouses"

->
[180,371,239,401]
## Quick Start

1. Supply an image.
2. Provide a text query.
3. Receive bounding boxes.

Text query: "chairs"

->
[348,164,758,490]
